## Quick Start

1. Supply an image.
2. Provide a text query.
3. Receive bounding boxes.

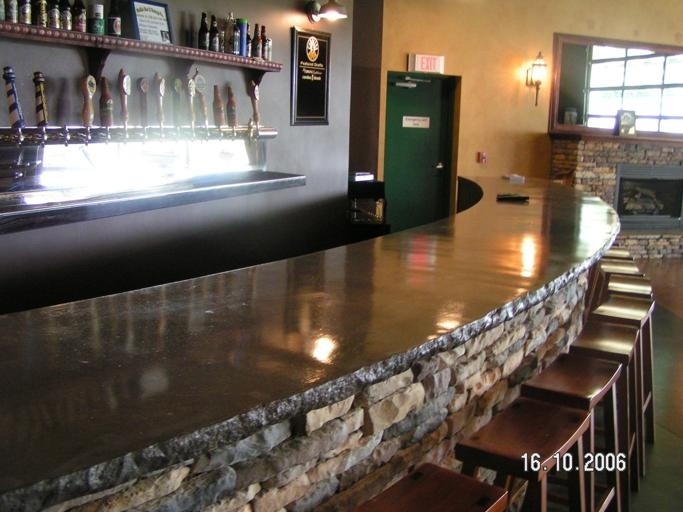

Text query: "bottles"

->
[99,77,113,126]
[563,107,577,126]
[197,10,272,59]
[4,1,122,37]
[226,87,238,128]
[210,84,225,127]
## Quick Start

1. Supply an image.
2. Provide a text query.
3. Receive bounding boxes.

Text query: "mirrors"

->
[551,31,683,137]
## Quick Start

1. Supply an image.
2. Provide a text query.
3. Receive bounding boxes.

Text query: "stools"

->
[520,351,624,511]
[570,318,640,505]
[590,294,655,478]
[454,396,592,512]
[351,462,509,512]
[583,248,655,323]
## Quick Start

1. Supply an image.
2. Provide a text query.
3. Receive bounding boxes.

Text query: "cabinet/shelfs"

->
[0,21,283,146]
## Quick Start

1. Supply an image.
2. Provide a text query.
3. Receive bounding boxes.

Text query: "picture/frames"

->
[129,0,174,45]
[290,25,331,126]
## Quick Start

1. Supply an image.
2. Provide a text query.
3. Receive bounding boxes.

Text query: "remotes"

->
[497,193,530,201]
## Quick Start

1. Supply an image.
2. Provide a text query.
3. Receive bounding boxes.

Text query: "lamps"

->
[305,0,347,25]
[525,52,548,107]
